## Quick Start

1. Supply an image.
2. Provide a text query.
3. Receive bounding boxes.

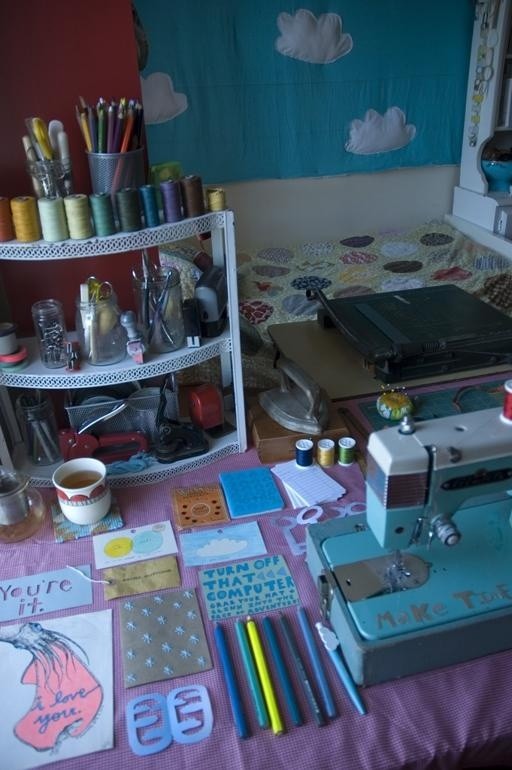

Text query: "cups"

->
[52,457,111,527]
[0,464,34,529]
[30,296,71,370]
[73,290,127,367]
[131,266,184,354]
[27,154,77,201]
[15,394,61,468]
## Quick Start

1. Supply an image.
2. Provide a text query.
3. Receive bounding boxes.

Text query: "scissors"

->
[85,276,114,359]
[131,262,175,346]
[20,395,36,418]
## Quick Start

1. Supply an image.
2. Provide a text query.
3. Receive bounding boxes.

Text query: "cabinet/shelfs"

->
[0,208,249,492]
[441,0,511,262]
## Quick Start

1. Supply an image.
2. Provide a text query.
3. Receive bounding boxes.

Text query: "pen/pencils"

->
[75,95,144,202]
[27,389,60,466]
[212,607,364,739]
[141,253,151,330]
[22,117,72,199]
